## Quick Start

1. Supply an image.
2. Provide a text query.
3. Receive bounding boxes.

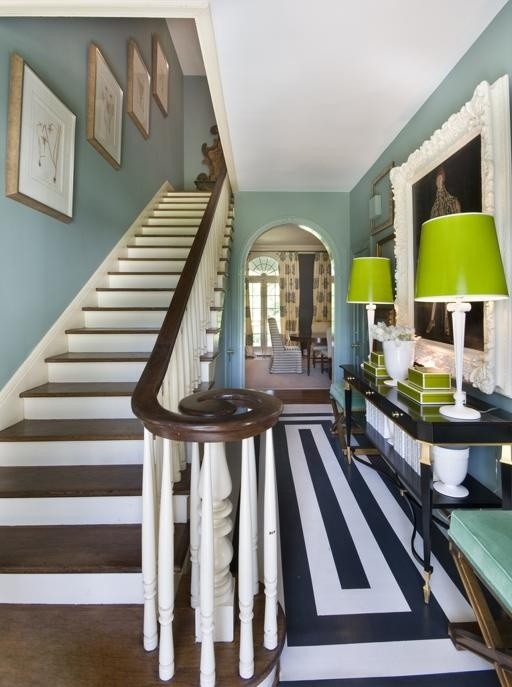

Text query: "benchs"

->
[443,506,512,686]
[329,382,378,455]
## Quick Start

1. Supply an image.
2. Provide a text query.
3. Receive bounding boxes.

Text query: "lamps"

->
[346,256,395,370]
[413,212,511,421]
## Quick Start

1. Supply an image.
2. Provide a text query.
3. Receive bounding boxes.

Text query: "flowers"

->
[369,320,423,342]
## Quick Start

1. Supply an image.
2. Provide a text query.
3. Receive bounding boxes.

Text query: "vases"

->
[382,341,417,386]
[432,445,470,499]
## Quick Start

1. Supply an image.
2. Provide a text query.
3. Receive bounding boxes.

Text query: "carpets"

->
[233,402,505,687]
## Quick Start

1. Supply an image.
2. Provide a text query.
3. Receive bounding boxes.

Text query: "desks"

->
[340,361,512,605]
[289,332,327,377]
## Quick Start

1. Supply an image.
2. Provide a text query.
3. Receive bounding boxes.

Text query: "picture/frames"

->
[127,36,153,140]
[7,50,82,225]
[389,73,512,395]
[368,160,394,236]
[151,35,171,119]
[86,42,125,172]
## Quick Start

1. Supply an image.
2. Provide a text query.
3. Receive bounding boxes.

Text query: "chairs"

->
[268,318,302,374]
[321,328,332,380]
